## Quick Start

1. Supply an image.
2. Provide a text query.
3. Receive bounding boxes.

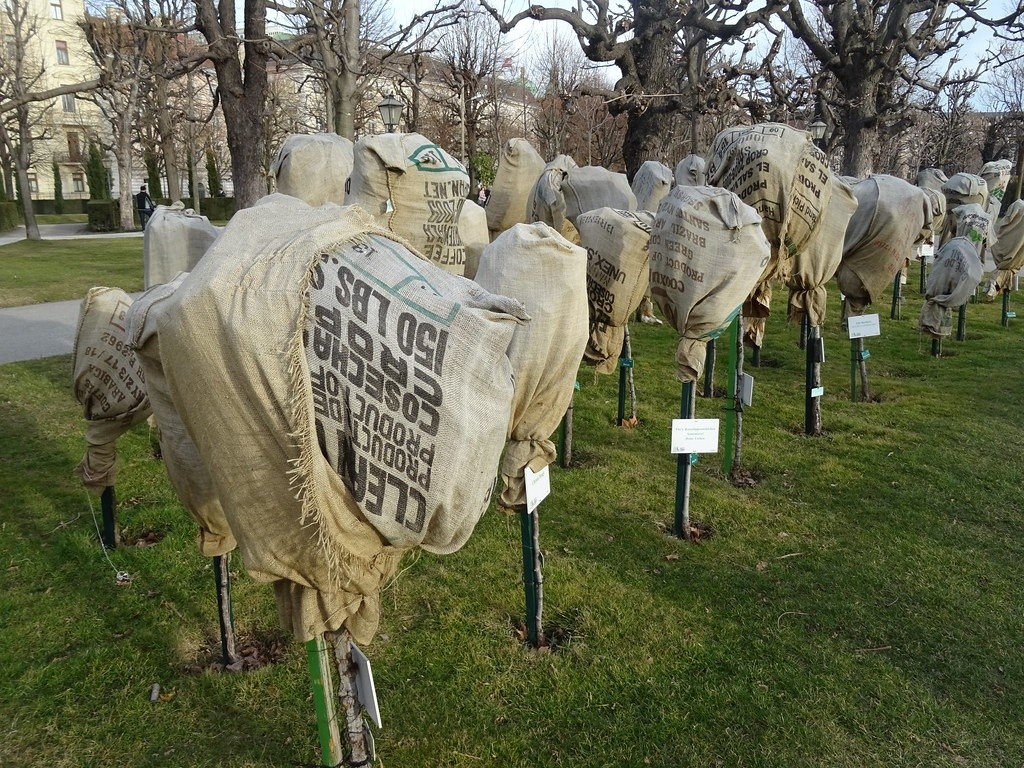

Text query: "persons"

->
[136,185,155,233]
[478,187,486,207]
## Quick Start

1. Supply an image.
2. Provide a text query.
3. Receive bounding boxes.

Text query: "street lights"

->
[377,90,405,132]
